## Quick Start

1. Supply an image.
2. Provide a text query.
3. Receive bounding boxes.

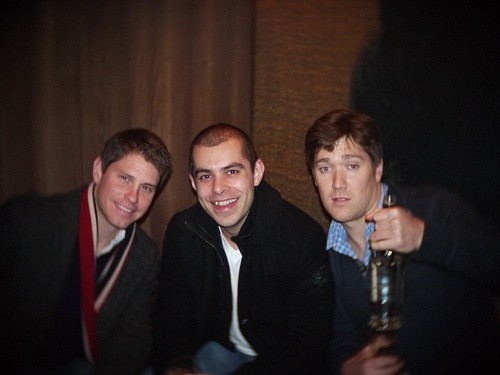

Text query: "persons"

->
[153,121,336,375]
[305,109,500,375]
[0,127,172,375]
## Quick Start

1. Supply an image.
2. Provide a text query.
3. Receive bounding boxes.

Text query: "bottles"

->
[369,194,407,332]
